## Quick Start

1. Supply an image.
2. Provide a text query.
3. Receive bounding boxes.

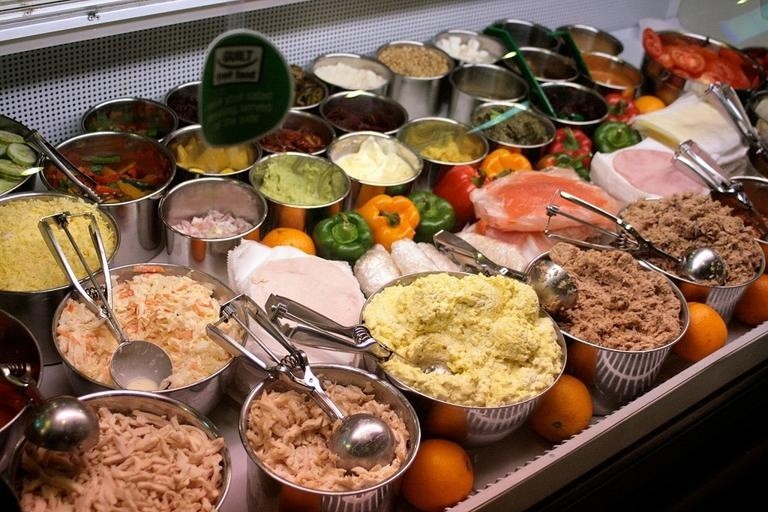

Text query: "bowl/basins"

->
[51,263,245,408]
[6,388,232,512]
[520,247,693,418]
[639,28,767,173]
[709,175,767,275]
[353,269,568,447]
[0,16,645,367]
[235,363,422,512]
[614,198,765,327]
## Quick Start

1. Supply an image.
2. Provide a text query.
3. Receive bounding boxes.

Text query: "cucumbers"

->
[0,129,37,195]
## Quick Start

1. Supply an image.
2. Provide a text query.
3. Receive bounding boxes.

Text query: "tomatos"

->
[641,26,759,91]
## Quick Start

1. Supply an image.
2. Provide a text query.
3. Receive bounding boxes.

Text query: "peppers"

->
[311,91,642,267]
